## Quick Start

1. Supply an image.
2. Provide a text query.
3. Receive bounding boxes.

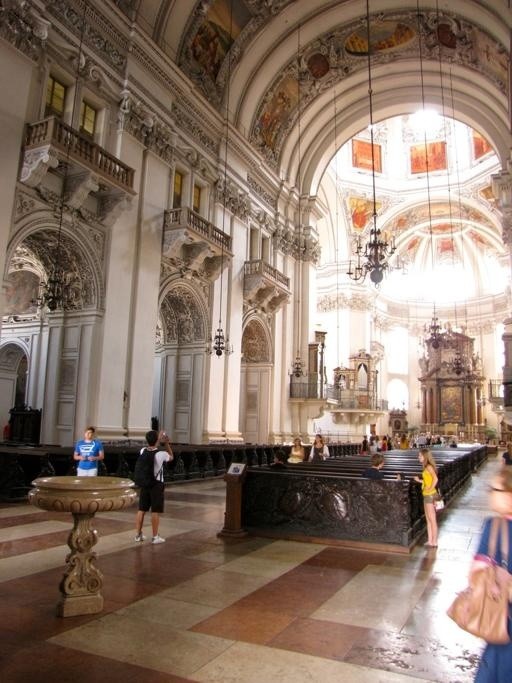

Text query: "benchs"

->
[216,442,488,554]
[2,442,361,505]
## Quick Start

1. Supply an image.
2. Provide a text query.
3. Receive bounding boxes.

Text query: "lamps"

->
[414,1,474,377]
[208,1,234,357]
[289,0,309,378]
[345,0,403,284]
[29,1,88,310]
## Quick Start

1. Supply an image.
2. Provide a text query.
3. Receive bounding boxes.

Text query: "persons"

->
[472,465,512,682]
[500,441,512,465]
[360,430,458,451]
[71,426,105,476]
[288,439,306,464]
[307,434,331,463]
[361,455,390,480]
[415,448,442,547]
[270,449,288,470]
[134,431,174,545]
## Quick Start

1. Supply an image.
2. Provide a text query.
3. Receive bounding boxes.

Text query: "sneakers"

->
[151,536,165,544]
[135,531,147,541]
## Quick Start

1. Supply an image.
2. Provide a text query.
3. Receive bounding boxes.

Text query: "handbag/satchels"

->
[433,495,444,512]
[445,565,510,645]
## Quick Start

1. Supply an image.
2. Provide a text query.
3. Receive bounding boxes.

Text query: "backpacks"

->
[133,449,161,487]
